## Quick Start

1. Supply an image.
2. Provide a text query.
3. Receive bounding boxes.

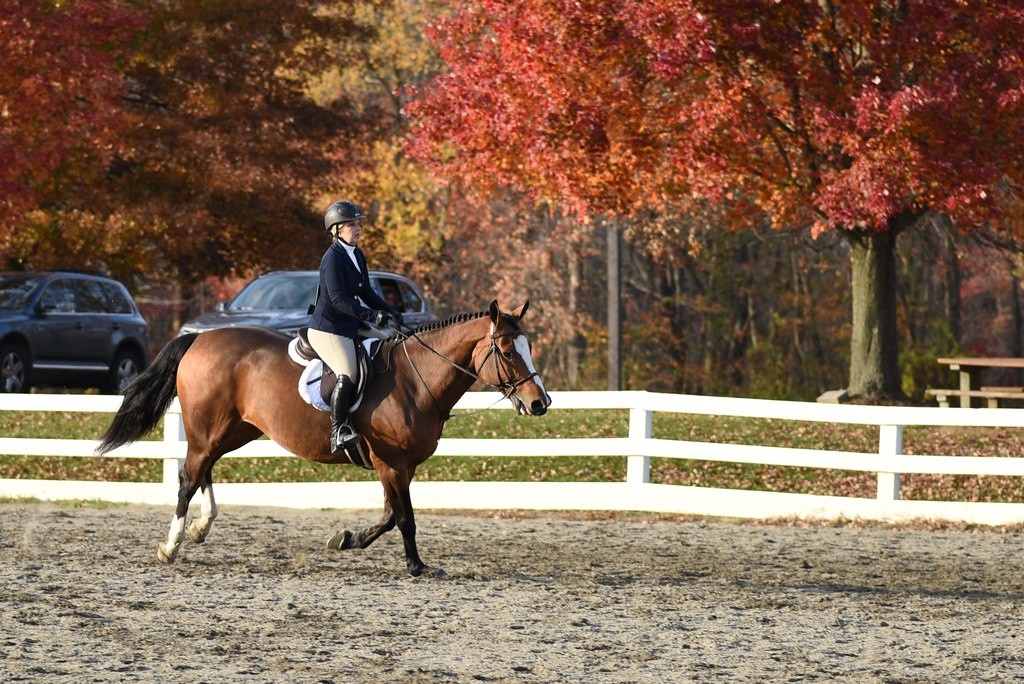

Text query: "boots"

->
[329,375,362,455]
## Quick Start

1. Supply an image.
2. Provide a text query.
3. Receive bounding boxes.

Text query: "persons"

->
[307,201,404,454]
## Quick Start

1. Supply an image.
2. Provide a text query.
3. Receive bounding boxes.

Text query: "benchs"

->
[925,388,1024,409]
[981,386,1024,407]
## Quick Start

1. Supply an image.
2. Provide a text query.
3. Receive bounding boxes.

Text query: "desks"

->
[938,358,1024,409]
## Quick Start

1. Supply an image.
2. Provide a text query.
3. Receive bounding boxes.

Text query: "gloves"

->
[382,304,404,325]
[369,309,390,328]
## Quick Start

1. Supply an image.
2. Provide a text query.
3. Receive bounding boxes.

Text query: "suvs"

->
[1,267,150,396]
[177,269,440,342]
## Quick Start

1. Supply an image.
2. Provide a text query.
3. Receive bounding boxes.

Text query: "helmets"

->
[324,200,366,231]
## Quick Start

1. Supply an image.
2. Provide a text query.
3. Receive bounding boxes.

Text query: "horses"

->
[93,297,552,579]
